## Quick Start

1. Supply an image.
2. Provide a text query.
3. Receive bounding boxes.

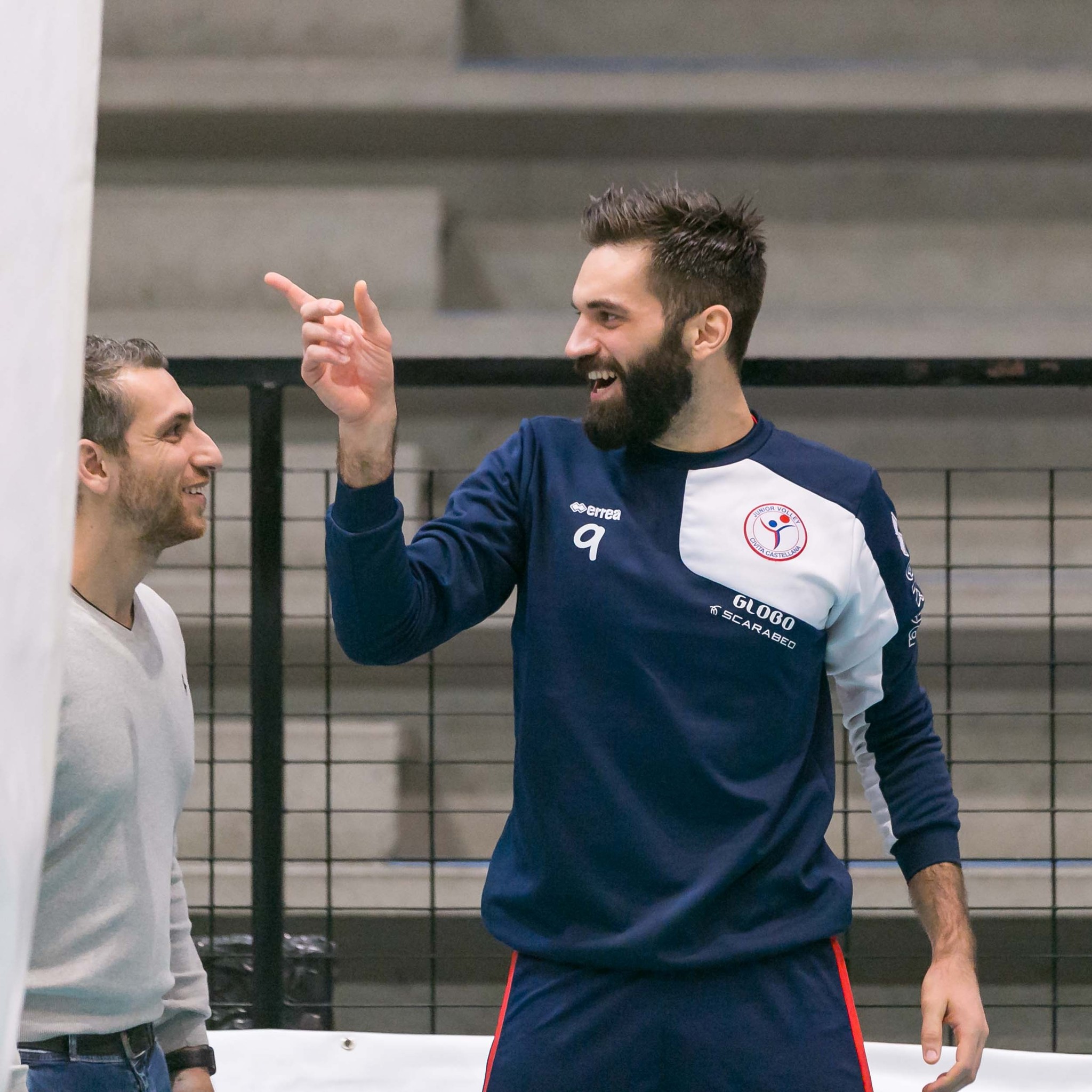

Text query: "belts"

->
[18,1021,154,1056]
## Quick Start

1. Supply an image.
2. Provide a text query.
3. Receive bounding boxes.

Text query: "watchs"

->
[165,1044,217,1078]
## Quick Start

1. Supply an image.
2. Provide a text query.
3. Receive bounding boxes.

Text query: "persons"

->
[18,332,225,1091]
[263,172,987,1092]
